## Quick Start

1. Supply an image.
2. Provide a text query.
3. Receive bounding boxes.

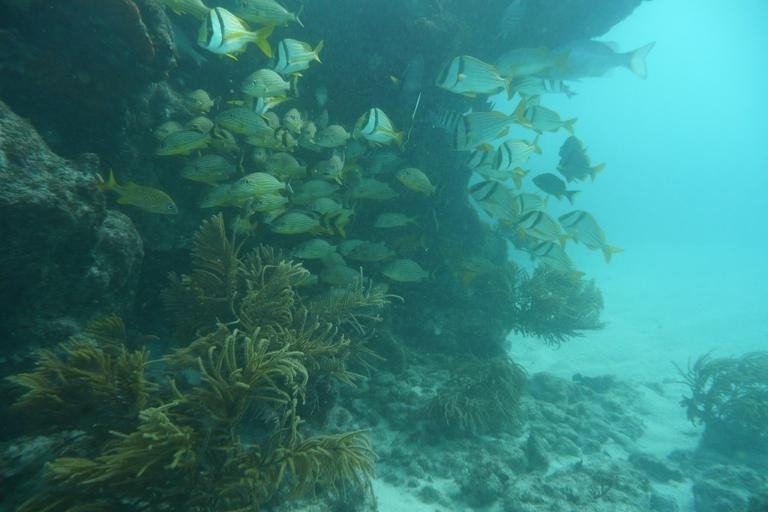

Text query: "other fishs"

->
[93,0,657,291]
